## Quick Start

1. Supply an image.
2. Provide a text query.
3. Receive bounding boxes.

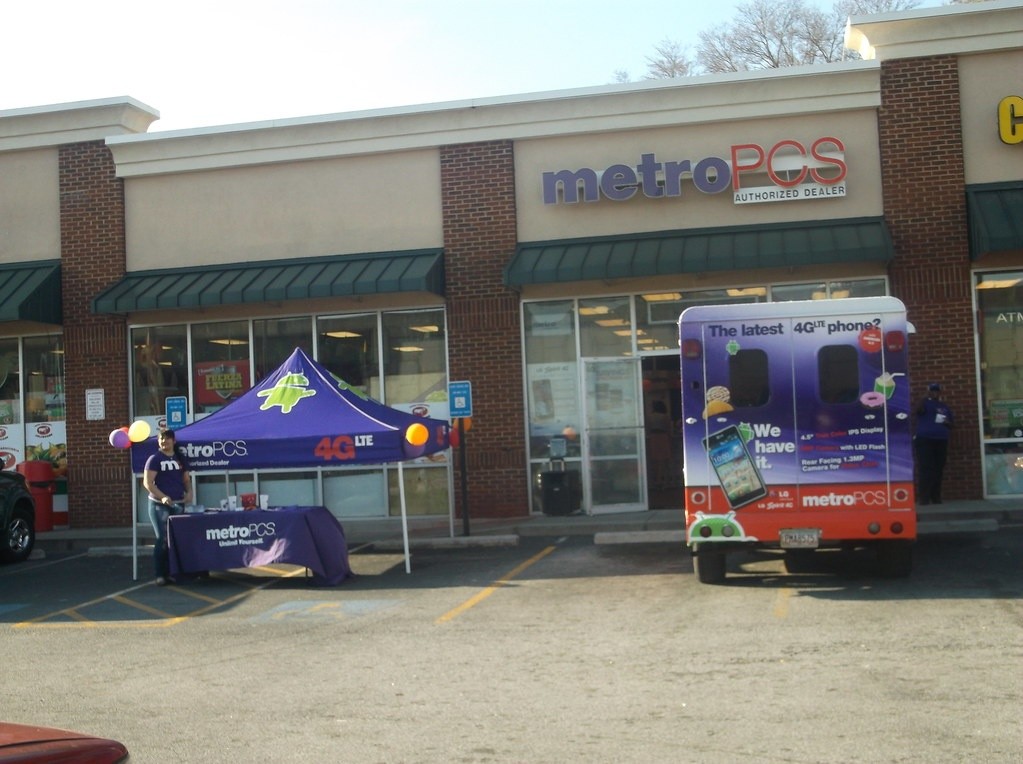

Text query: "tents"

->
[129,346,456,582]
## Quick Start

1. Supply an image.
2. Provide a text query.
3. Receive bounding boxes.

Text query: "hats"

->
[926,383,940,391]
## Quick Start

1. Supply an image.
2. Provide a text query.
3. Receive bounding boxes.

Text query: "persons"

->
[914,383,954,505]
[142,430,193,586]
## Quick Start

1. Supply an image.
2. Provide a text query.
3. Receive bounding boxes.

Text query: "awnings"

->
[966,180,1023,263]
[503,214,892,297]
[0,258,62,325]
[90,246,448,320]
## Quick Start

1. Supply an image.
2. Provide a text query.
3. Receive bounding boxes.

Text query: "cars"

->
[0,458,36,563]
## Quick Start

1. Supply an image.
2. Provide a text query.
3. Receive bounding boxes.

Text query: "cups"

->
[220,499,228,511]
[260,495,269,510]
[228,496,237,511]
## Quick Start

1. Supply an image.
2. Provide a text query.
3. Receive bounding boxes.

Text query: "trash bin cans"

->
[17,461,54,532]
[537,469,580,516]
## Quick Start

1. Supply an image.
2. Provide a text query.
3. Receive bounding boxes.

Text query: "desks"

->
[168,506,329,583]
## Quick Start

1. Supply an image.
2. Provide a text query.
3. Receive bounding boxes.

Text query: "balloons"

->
[447,417,471,447]
[563,428,576,441]
[403,440,425,457]
[405,423,428,445]
[108,420,150,447]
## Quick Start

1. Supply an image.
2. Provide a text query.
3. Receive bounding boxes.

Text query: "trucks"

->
[679,296,916,583]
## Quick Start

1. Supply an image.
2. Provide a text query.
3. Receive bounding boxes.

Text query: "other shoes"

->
[156,577,165,586]
[919,495,930,505]
[931,491,941,503]
